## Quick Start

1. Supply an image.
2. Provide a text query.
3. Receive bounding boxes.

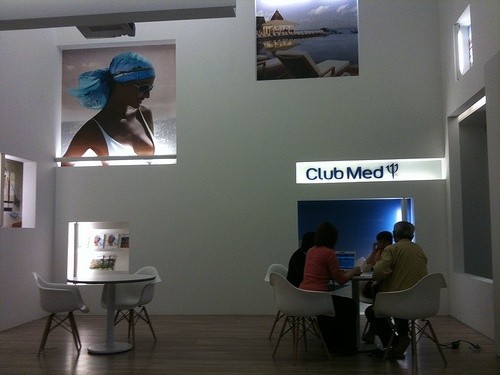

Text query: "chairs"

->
[264,263,318,342]
[257,49,349,81]
[32,271,89,357]
[268,272,335,367]
[374,269,448,375]
[101,265,162,350]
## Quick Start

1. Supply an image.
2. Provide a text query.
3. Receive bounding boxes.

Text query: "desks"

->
[67,272,156,354]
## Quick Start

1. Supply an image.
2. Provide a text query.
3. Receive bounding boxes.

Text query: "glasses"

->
[131,82,153,98]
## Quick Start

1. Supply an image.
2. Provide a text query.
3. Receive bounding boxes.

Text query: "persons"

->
[362,221,428,358]
[287,222,362,357]
[62,52,157,166]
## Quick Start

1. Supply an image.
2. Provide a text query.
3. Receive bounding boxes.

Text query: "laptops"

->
[335,250,356,272]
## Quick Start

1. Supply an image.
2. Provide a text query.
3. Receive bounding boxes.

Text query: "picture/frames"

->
[117,234,130,249]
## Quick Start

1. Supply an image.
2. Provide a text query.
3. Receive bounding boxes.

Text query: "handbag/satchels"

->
[366,280,385,297]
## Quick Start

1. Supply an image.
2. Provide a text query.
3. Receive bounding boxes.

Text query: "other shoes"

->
[363,329,375,344]
[386,335,409,359]
